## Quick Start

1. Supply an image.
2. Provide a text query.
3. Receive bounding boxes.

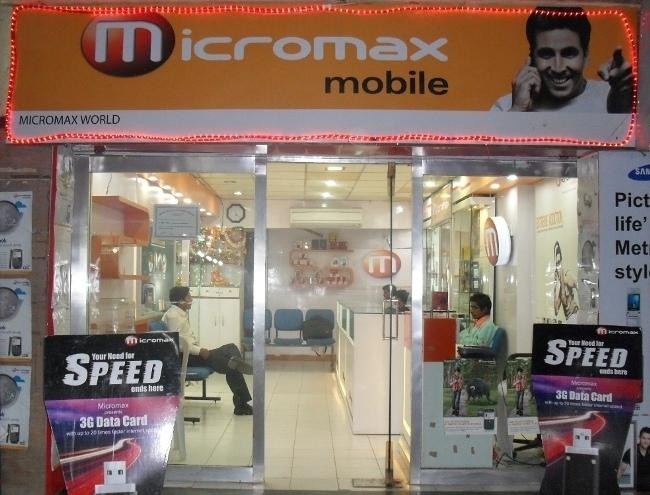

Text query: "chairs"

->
[243,308,336,357]
[457,327,504,362]
[149,319,221,425]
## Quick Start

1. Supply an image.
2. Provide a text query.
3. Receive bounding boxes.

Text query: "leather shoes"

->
[228,359,253,375]
[234,404,253,415]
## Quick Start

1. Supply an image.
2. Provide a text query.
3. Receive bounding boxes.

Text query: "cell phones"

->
[624,288,640,326]
[9,248,22,270]
[9,336,22,356]
[6,424,20,444]
[483,408,494,428]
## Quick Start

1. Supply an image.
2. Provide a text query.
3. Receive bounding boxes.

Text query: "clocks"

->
[226,203,246,223]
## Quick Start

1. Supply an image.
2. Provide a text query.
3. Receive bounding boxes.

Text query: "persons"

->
[383,284,410,313]
[487,5,633,115]
[618,425,650,494]
[160,284,254,416]
[449,368,464,417]
[513,367,529,416]
[553,242,578,325]
[453,291,505,357]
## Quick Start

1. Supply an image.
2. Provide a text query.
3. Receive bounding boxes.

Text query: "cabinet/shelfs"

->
[90,242,166,321]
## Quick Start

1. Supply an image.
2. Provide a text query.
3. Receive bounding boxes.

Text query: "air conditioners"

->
[288,207,363,230]
